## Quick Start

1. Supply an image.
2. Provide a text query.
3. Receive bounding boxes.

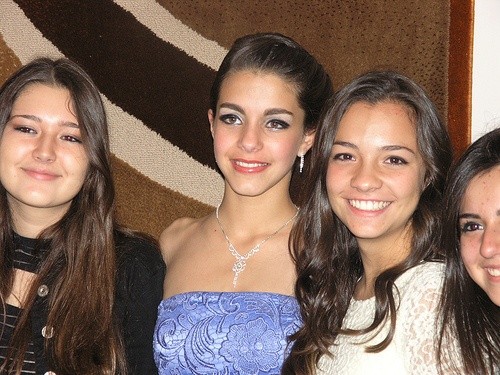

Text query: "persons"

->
[280,70,500,375]
[159,32,334,375]
[0,58,166,375]
[433,127,500,375]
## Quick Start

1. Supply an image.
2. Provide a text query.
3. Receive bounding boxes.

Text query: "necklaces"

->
[216,203,299,287]
